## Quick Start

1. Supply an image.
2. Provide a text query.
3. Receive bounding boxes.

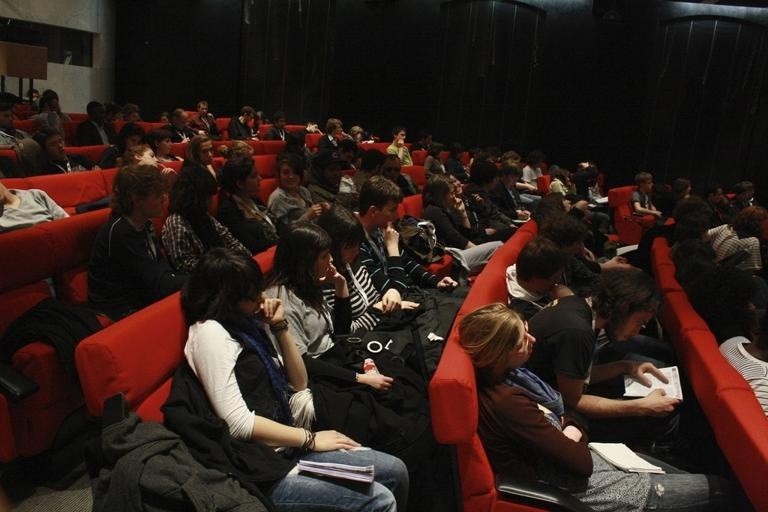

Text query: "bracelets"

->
[270,319,289,334]
[302,427,316,452]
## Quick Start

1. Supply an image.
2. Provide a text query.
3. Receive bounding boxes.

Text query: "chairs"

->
[1,100,43,166]
[74,292,185,425]
[25,171,107,220]
[652,236,668,251]
[0,227,114,502]
[517,220,538,239]
[102,169,118,195]
[609,185,655,245]
[57,113,162,161]
[34,208,113,308]
[152,111,470,282]
[655,266,684,295]
[711,389,768,510]
[501,231,533,255]
[651,246,674,269]
[458,273,507,316]
[481,246,519,278]
[536,163,551,195]
[664,291,709,358]
[428,316,599,512]
[684,331,754,430]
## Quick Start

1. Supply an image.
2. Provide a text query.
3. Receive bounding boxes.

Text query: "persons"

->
[521,152,543,187]
[0,156,16,177]
[128,144,179,177]
[353,148,387,193]
[161,167,253,273]
[68,101,110,146]
[0,179,70,233]
[383,154,422,197]
[353,147,365,172]
[159,111,169,121]
[122,104,141,121]
[147,128,184,163]
[459,302,741,512]
[28,96,60,121]
[181,135,216,185]
[468,146,482,168]
[374,129,384,143]
[387,125,413,168]
[353,176,458,296]
[564,219,634,274]
[446,141,470,184]
[668,239,718,291]
[488,146,499,163]
[98,123,146,168]
[701,183,726,227]
[0,92,32,145]
[218,139,254,160]
[480,153,493,164]
[318,118,342,151]
[570,162,610,210]
[348,126,363,144]
[303,120,324,135]
[26,128,99,178]
[529,267,695,467]
[689,265,768,345]
[267,153,332,226]
[631,171,663,218]
[547,165,610,234]
[186,101,214,138]
[506,236,575,319]
[181,248,409,512]
[337,139,357,170]
[103,104,123,124]
[307,150,360,212]
[588,177,606,204]
[264,110,289,140]
[265,221,425,473]
[679,197,713,232]
[423,174,506,276]
[444,172,500,245]
[277,132,312,170]
[160,108,197,144]
[363,130,376,144]
[741,181,753,207]
[319,206,420,335]
[409,130,434,154]
[719,308,768,419]
[87,163,190,321]
[489,160,531,227]
[502,152,542,215]
[702,206,765,273]
[228,106,260,142]
[43,89,72,122]
[655,178,691,218]
[22,88,40,105]
[531,192,596,263]
[540,212,599,293]
[425,142,448,179]
[730,182,747,213]
[464,159,518,237]
[663,181,673,192]
[497,151,502,162]
[217,156,280,255]
[337,127,352,139]
[249,111,270,136]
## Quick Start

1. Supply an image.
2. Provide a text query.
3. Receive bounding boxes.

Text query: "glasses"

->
[508,319,529,357]
[240,289,261,303]
